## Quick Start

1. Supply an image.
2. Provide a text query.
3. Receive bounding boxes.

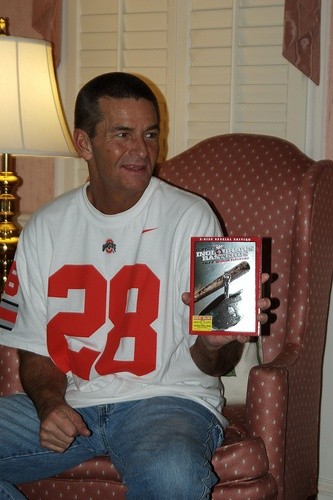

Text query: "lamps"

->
[0,18,81,296]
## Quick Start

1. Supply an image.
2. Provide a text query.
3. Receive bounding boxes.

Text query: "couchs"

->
[0,132,333,500]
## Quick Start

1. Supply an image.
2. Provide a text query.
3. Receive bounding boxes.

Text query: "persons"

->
[0,73,271,500]
